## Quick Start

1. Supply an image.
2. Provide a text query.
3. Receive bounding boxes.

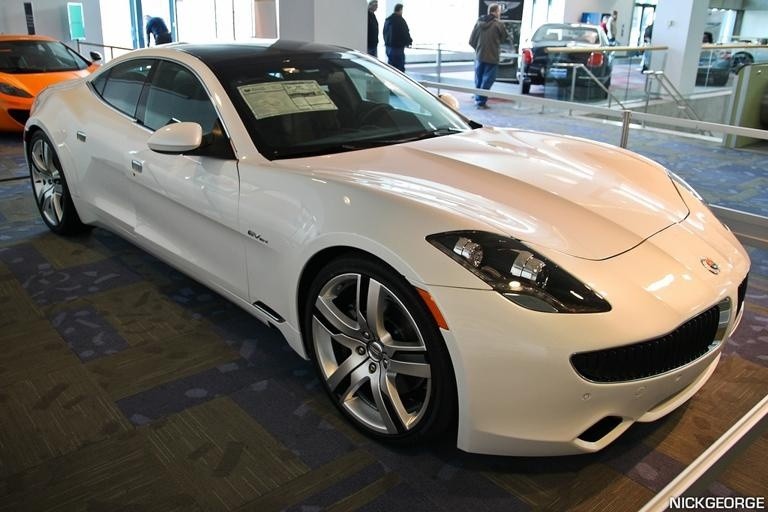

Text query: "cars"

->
[516,21,617,99]
[730,47,768,77]
[640,47,735,88]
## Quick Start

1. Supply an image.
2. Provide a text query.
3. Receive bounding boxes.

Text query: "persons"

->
[606,10,618,47]
[367,0,380,58]
[468,4,508,111]
[599,16,607,35]
[383,3,414,73]
[143,12,172,48]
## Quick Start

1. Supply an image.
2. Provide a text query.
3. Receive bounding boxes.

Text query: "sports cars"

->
[23,38,753,470]
[0,33,103,138]
